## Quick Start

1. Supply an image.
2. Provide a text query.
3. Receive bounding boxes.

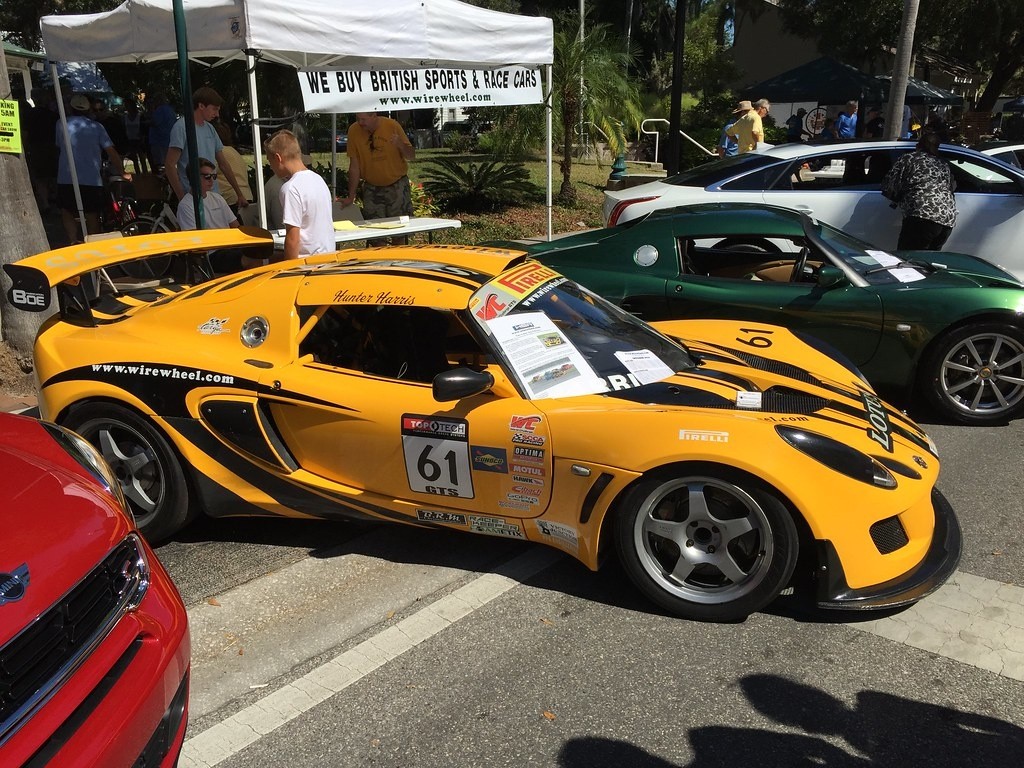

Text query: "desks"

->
[269,216,462,251]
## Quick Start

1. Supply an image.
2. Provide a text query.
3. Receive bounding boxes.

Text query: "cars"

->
[309,126,348,153]
[601,140,1024,285]
[951,143,1024,184]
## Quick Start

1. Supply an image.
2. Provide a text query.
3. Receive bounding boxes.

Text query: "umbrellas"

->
[740,56,963,139]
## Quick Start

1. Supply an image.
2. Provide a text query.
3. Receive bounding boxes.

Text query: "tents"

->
[1003,97,1024,112]
[40,0,554,267]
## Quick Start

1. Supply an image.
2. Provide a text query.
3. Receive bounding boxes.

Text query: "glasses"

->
[94,107,104,112]
[200,173,217,180]
[368,134,374,151]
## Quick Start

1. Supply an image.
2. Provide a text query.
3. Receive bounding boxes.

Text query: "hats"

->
[731,100,754,114]
[70,95,90,110]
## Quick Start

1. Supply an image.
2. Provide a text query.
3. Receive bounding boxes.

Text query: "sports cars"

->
[2,226,964,623]
[0,412,193,768]
[471,199,1024,428]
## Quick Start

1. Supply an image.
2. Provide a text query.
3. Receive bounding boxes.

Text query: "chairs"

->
[333,200,365,222]
[236,203,260,227]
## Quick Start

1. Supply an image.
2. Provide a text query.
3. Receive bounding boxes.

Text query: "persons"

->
[12,86,177,217]
[166,86,249,209]
[926,112,948,142]
[786,108,812,144]
[265,153,312,229]
[908,109,921,140]
[56,95,132,246]
[336,112,415,248]
[718,101,754,160]
[727,99,770,155]
[264,130,336,260]
[214,123,254,226]
[883,133,956,252]
[820,118,835,141]
[177,157,240,275]
[866,109,885,141]
[834,101,858,143]
[900,105,911,141]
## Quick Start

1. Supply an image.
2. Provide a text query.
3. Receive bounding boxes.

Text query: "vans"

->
[438,118,497,153]
